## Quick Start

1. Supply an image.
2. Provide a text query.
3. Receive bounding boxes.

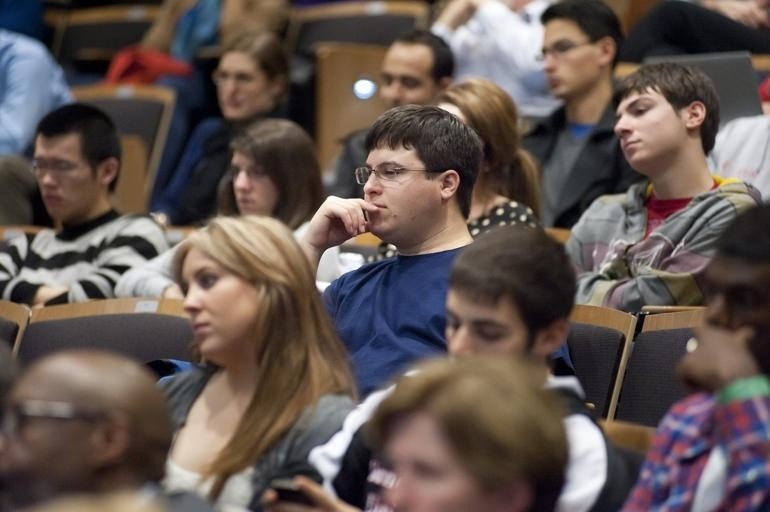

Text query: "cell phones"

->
[267,475,310,506]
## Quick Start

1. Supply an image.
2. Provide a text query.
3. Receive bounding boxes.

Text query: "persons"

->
[428,1,770,313]
[613,198,769,511]
[288,32,575,409]
[2,1,353,511]
[247,224,608,512]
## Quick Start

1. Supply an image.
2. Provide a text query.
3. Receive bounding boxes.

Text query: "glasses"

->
[352,163,445,185]
